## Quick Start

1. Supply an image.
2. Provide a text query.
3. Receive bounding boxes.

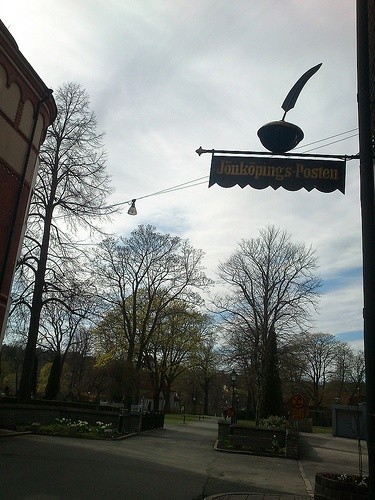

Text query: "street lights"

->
[228,369,238,425]
